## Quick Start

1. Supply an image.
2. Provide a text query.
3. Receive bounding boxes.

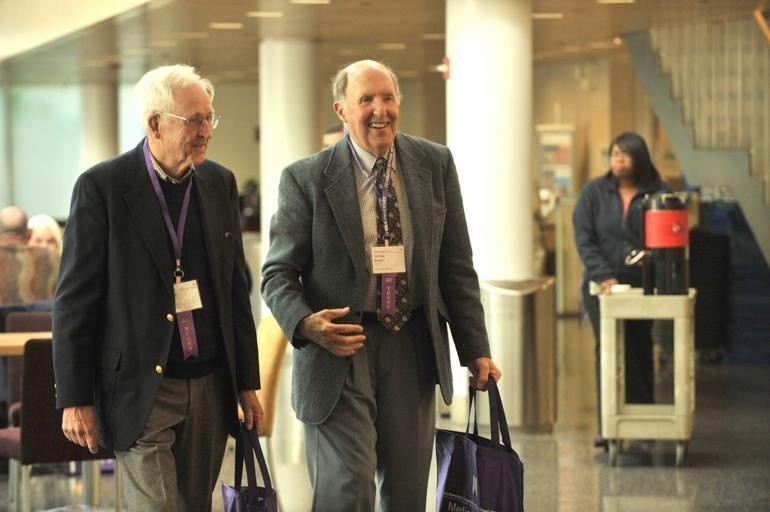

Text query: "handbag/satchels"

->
[221,421,277,511]
[433,376,524,512]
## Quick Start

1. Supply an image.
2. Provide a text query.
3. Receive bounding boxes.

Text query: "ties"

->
[372,156,412,336]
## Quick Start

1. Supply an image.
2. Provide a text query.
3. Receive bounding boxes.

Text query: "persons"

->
[260,60,502,512]
[52,64,264,512]
[572,133,673,451]
[27,215,62,251]
[0,206,61,304]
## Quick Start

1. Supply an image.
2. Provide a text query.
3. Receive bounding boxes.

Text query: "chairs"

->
[5,312,52,405]
[237,315,288,512]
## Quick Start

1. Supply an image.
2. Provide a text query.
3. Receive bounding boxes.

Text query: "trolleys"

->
[597,283,702,466]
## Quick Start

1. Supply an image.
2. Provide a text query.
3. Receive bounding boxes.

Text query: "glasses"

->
[156,110,222,131]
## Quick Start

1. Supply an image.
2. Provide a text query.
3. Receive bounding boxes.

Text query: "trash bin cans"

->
[475,276,557,434]
